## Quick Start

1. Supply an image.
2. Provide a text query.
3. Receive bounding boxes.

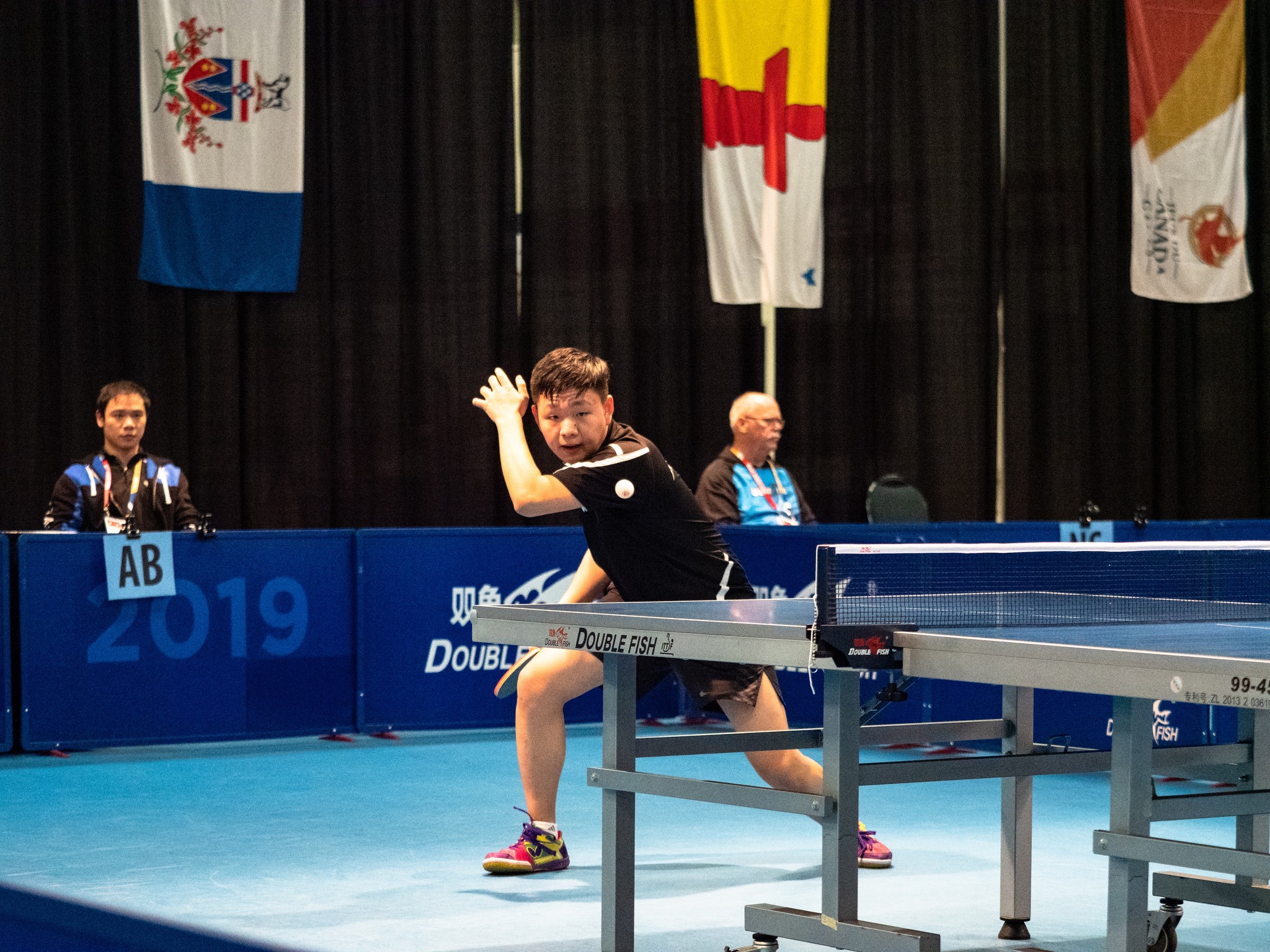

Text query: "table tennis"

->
[614,479,635,499]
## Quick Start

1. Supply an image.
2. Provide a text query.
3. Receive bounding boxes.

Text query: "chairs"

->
[866,473,929,524]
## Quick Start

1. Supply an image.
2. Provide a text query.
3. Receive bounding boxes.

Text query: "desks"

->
[471,538,1270,952]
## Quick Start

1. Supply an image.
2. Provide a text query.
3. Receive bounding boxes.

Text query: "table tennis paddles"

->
[493,647,542,699]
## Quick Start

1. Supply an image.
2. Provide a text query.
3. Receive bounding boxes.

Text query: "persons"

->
[44,380,203,535]
[696,392,821,525]
[472,348,893,871]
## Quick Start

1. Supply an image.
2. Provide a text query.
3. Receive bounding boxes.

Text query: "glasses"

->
[746,417,786,430]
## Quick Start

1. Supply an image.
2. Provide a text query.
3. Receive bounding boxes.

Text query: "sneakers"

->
[855,822,892,868]
[483,807,569,873]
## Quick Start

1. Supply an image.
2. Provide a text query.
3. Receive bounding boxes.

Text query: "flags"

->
[137,0,305,291]
[696,0,830,309]
[1125,0,1254,305]
